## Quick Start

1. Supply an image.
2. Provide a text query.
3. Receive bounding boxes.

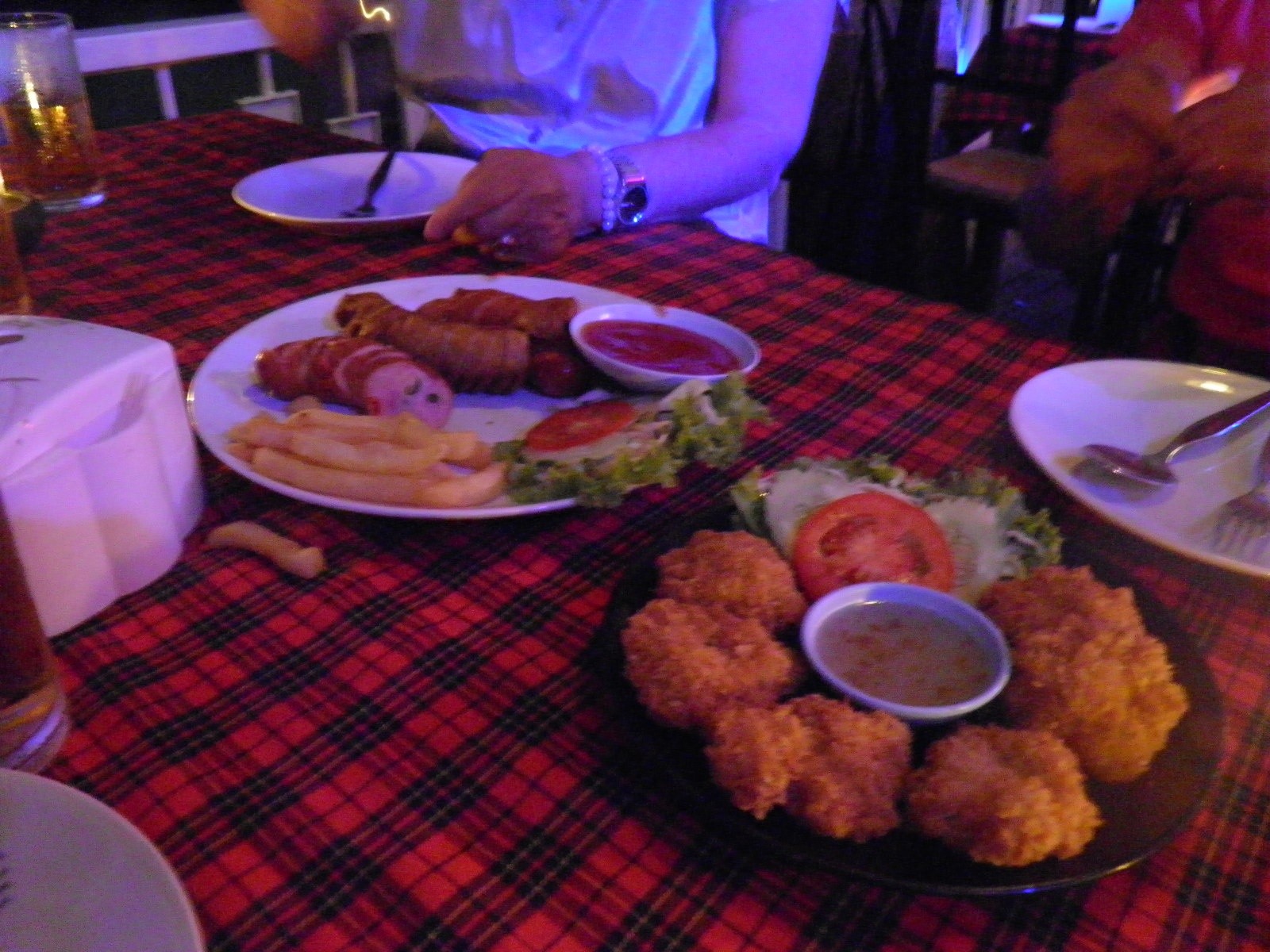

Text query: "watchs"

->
[604,150,651,227]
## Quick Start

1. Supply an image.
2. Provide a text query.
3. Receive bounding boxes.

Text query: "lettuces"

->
[720,453,1067,586]
[491,372,772,507]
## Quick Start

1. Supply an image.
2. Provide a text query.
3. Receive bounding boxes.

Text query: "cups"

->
[0,499,71,778]
[0,175,34,318]
[1,11,108,210]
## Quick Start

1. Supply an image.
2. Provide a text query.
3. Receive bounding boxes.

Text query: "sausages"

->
[254,287,587,427]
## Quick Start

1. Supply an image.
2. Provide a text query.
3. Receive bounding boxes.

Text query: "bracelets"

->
[582,142,619,231]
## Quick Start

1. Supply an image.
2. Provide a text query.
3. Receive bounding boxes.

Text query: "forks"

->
[1217,439,1270,557]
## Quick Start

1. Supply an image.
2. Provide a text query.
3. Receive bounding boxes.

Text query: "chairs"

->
[912,0,1190,355]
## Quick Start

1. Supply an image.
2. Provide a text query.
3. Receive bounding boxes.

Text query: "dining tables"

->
[0,106,1270,952]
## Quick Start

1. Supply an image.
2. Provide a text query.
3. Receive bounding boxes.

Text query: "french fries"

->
[201,399,509,577]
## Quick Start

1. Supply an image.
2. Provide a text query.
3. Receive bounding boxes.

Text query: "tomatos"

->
[524,399,638,449]
[793,492,953,598]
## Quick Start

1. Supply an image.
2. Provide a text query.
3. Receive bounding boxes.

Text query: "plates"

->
[1008,358,1270,582]
[0,769,206,952]
[802,583,1010,718]
[230,152,484,238]
[570,304,761,390]
[185,273,763,519]
[599,499,1227,895]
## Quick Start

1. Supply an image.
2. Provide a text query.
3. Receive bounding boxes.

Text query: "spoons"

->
[1084,391,1270,486]
[342,146,399,218]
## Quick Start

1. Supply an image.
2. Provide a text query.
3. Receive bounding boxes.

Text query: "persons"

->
[1046,1,1270,384]
[245,0,837,265]
